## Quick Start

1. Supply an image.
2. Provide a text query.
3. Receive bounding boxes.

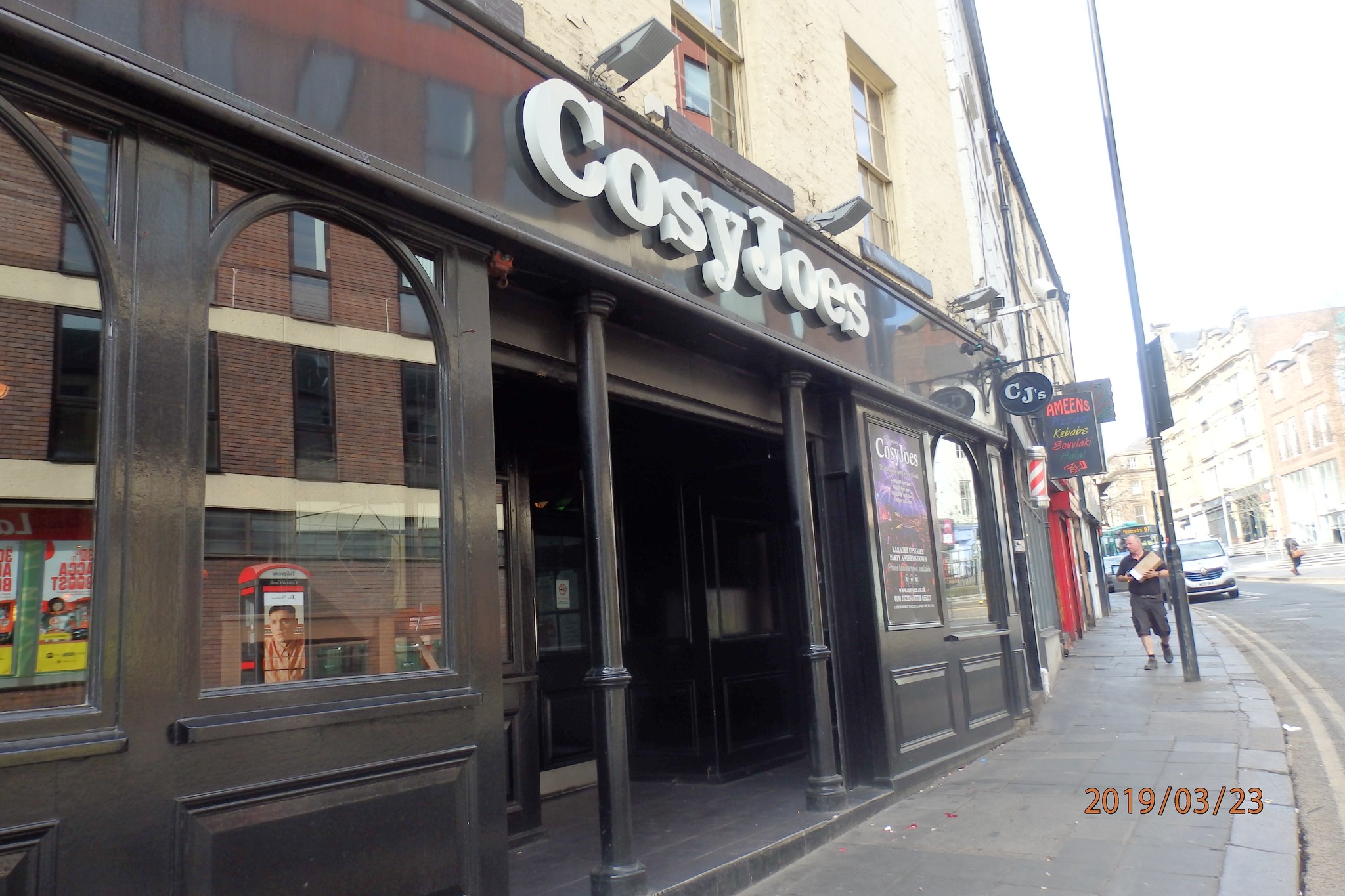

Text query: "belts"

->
[1141,595,1156,598]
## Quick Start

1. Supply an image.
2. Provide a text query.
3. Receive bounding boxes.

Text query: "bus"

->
[1099,521,1163,586]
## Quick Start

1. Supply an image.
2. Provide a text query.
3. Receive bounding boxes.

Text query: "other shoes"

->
[1291,568,1300,575]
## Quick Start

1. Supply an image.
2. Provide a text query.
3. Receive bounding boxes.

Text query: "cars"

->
[1161,538,1238,603]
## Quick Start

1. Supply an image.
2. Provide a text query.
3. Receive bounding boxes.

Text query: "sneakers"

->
[1144,657,1158,670]
[1161,642,1173,663]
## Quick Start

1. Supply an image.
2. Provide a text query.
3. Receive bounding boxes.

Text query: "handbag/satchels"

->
[1292,549,1304,558]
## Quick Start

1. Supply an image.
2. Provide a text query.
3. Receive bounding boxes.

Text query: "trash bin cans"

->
[395,640,445,673]
[313,646,344,677]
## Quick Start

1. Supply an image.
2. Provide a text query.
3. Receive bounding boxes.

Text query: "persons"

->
[264,605,305,683]
[1119,539,1126,552]
[41,598,74,630]
[1117,535,1173,670]
[1284,535,1301,575]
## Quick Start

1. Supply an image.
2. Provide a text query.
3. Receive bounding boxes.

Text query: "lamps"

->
[590,17,682,94]
[947,285,1000,314]
[803,195,873,239]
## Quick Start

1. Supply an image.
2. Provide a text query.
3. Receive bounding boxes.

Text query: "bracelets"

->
[1157,571,1160,577]
